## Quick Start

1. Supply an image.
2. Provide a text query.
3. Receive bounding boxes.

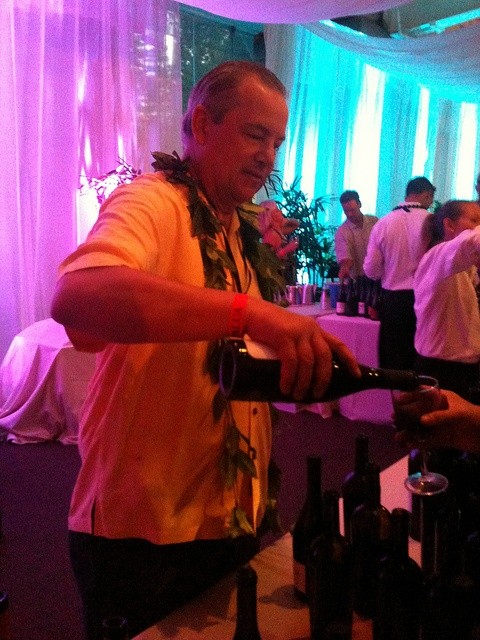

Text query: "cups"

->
[284,285,300,303]
[319,290,331,311]
[327,283,341,308]
[302,285,314,304]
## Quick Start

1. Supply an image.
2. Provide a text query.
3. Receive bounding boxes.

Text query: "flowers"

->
[243,194,304,262]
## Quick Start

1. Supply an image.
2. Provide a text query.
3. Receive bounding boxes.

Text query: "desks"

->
[132,451,429,639]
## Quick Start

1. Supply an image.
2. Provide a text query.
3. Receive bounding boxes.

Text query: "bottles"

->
[232,568,263,640]
[453,386,479,493]
[341,433,380,540]
[373,508,422,639]
[346,279,356,316]
[336,278,346,315]
[372,279,383,308]
[291,453,324,601]
[354,462,391,619]
[367,279,374,307]
[354,277,367,316]
[219,335,420,403]
[464,493,480,631]
[306,489,353,640]
[422,520,462,640]
[407,449,422,543]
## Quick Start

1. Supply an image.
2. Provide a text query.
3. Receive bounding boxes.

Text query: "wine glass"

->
[391,375,449,497]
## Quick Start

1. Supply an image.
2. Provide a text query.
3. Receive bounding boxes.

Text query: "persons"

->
[335,191,381,315]
[389,384,480,453]
[414,200,480,393]
[362,177,436,364]
[50,60,362,639]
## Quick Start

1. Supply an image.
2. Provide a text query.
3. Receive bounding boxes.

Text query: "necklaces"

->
[392,204,426,210]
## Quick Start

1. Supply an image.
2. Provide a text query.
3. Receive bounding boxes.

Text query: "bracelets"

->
[230,292,248,338]
